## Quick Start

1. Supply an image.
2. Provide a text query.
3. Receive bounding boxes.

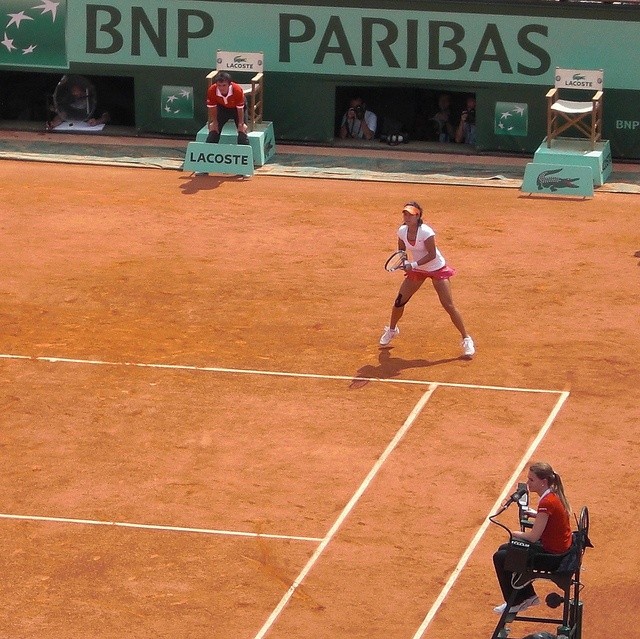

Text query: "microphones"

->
[490,484,536,550]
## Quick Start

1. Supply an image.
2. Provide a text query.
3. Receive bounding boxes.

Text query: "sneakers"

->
[493,601,528,613]
[236,174,251,178]
[380,324,400,345]
[523,595,541,607]
[461,335,475,356]
[194,171,209,176]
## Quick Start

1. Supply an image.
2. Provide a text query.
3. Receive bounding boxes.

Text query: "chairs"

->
[491,506,589,639]
[207,50,265,132]
[547,68,603,152]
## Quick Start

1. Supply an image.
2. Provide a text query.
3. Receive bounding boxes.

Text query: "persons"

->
[46,85,112,127]
[340,98,378,139]
[380,202,475,356]
[195,72,250,177]
[455,93,476,144]
[494,463,572,613]
[427,92,458,144]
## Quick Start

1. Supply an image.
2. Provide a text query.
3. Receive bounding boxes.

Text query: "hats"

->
[402,204,421,215]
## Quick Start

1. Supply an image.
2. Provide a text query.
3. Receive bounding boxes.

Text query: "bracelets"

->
[411,262,418,268]
[403,261,410,266]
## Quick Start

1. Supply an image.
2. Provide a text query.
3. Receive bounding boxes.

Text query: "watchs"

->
[238,123,245,127]
